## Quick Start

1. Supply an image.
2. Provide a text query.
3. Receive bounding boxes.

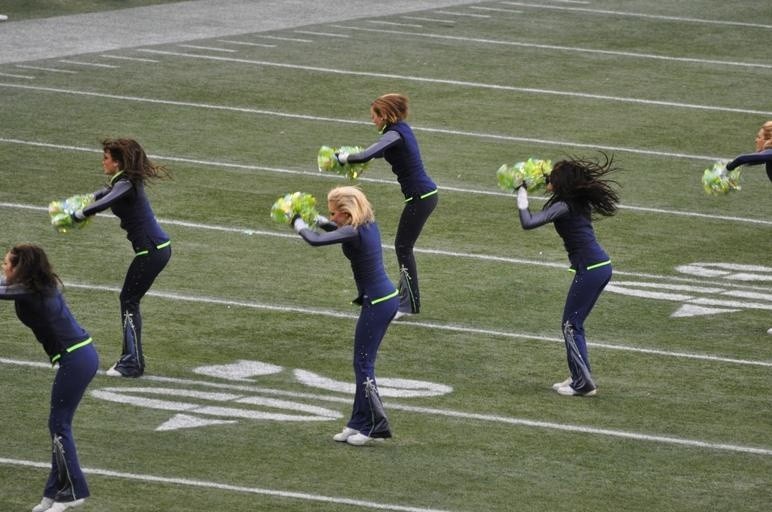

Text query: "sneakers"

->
[347,433,385,445]
[333,427,358,441]
[31,497,54,512]
[552,376,574,390]
[45,499,84,512]
[105,360,123,377]
[558,385,596,397]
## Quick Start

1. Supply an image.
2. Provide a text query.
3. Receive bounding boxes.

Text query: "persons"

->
[514,150,623,400]
[0,243,100,512]
[288,185,400,447]
[63,138,172,378]
[335,93,439,319]
[724,120,772,336]
